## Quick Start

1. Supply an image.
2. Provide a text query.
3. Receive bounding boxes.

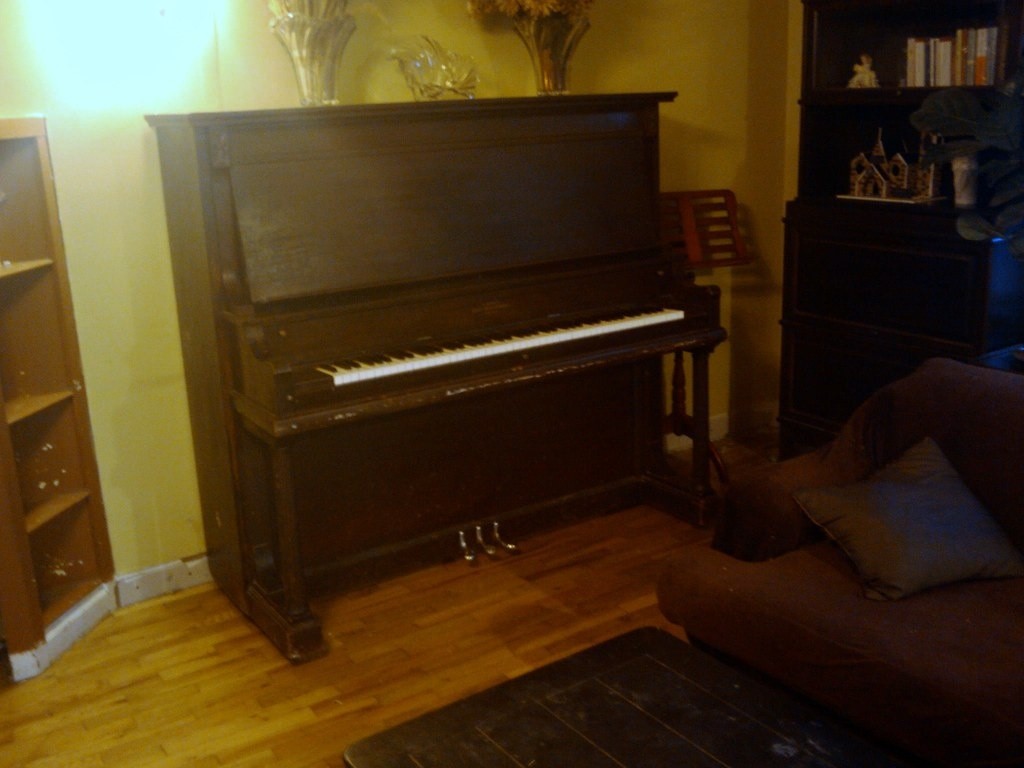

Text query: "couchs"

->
[656,356,1024,768]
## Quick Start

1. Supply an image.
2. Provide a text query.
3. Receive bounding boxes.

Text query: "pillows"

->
[796,436,1023,600]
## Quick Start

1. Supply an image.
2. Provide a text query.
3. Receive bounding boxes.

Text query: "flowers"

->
[469,0,598,28]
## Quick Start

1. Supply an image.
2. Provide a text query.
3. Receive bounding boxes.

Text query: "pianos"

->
[145,91,734,666]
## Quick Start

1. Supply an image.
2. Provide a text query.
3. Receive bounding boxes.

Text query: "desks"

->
[342,625,933,768]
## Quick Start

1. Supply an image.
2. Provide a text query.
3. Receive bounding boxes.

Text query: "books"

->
[908,25,995,88]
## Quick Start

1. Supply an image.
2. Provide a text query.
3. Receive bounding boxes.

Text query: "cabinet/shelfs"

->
[777,0,1024,445]
[1,118,115,681]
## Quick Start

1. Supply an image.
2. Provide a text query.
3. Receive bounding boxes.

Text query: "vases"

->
[516,15,592,96]
[267,0,357,105]
